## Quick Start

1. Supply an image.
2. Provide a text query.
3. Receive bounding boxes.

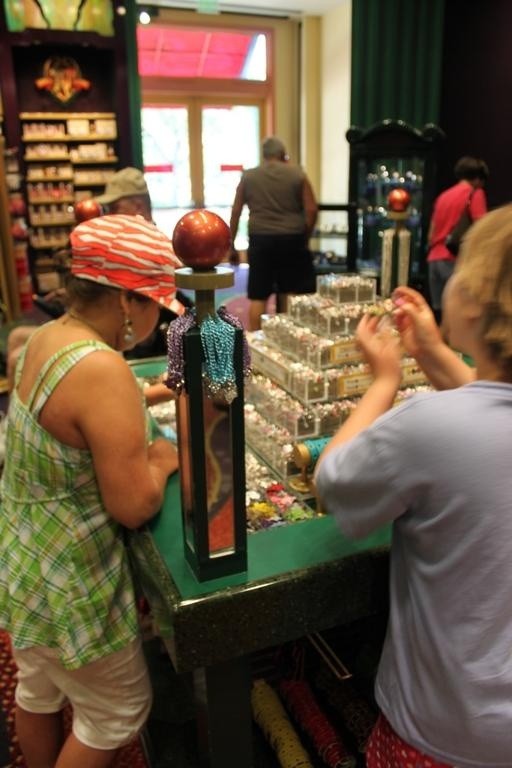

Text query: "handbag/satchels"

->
[443,206,474,259]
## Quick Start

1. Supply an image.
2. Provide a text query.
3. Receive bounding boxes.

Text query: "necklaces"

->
[163,312,194,395]
[196,313,240,406]
[216,306,253,377]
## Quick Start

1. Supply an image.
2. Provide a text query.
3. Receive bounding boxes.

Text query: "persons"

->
[424,154,489,326]
[6,165,156,396]
[0,213,180,768]
[310,201,512,768]
[227,137,320,331]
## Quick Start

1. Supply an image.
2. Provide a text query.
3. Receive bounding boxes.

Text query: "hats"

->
[94,166,149,203]
[69,214,185,317]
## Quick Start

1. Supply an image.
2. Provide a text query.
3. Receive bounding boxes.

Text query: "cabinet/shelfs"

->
[17,106,131,304]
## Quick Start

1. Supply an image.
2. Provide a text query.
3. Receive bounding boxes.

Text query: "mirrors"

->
[344,120,444,298]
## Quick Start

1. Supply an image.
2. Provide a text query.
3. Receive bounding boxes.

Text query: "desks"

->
[124,355,391,765]
